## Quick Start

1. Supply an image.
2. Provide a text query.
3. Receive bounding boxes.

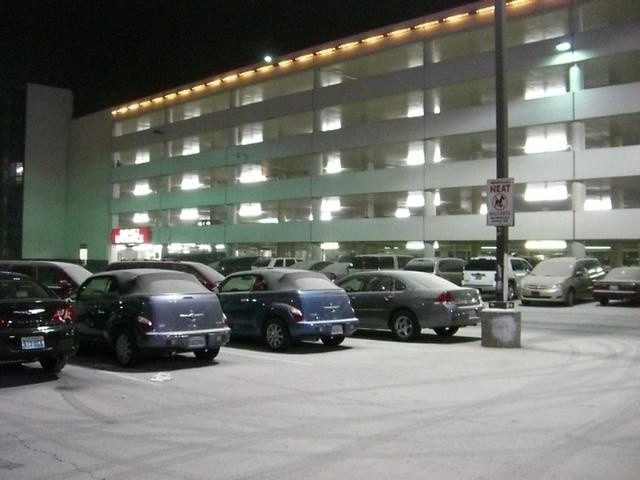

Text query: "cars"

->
[334,270,485,340]
[212,267,359,352]
[593,265,640,305]
[68,268,231,367]
[0,271,79,373]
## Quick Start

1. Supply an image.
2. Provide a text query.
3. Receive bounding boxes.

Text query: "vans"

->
[462,254,533,301]
[519,257,606,306]
